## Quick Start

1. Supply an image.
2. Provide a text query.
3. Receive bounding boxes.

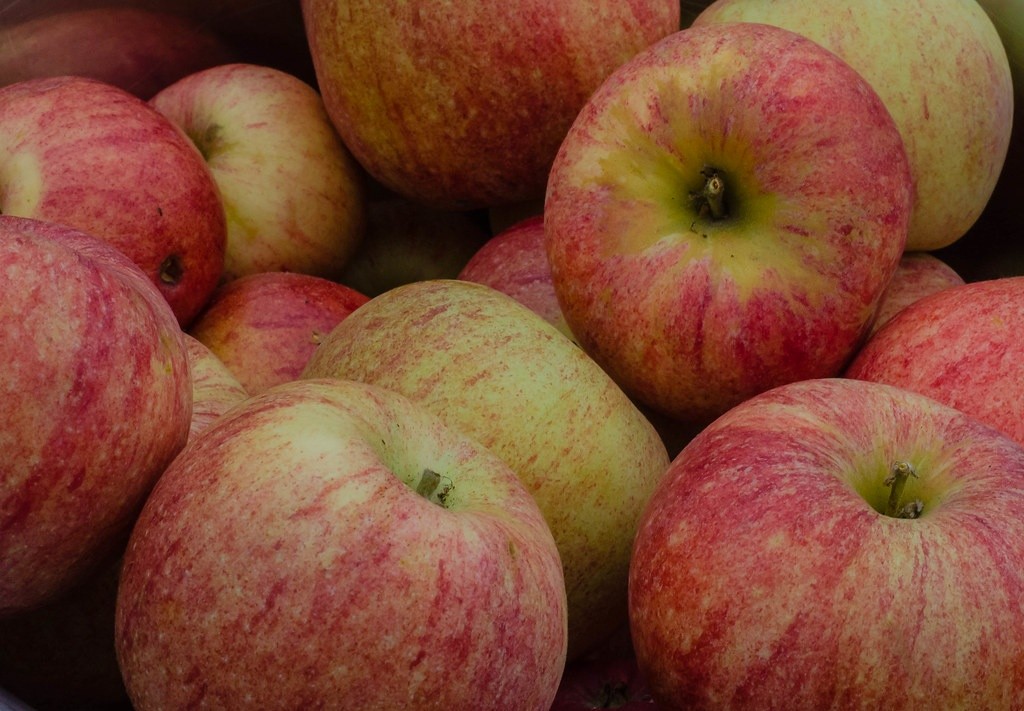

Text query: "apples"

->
[0,0,1024,711]
